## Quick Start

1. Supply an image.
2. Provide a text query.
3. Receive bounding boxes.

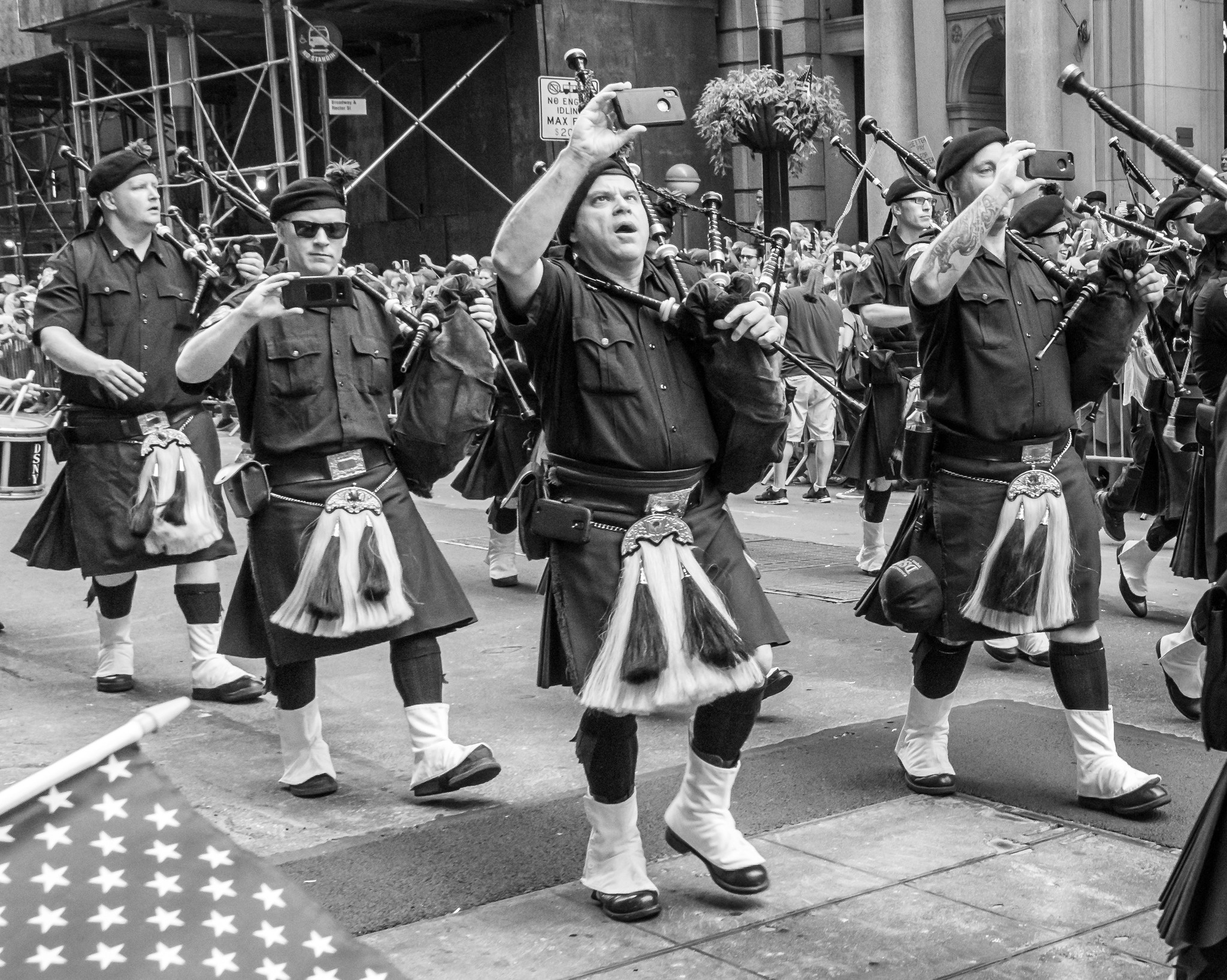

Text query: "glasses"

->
[1175,212,1200,224]
[740,255,758,260]
[283,220,350,240]
[1040,229,1071,244]
[897,197,937,206]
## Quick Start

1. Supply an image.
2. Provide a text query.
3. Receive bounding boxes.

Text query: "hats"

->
[268,158,361,222]
[936,126,1012,192]
[1085,191,1107,205]
[87,137,157,197]
[1154,187,1203,230]
[690,249,710,264]
[0,273,20,285]
[886,176,931,207]
[1008,181,1066,239]
[1194,200,1227,237]
[451,254,478,270]
[558,141,634,245]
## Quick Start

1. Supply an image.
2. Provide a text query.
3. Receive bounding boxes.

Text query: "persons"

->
[0,263,60,417]
[174,176,501,797]
[648,172,937,699]
[347,247,547,588]
[32,142,269,704]
[874,127,1173,815]
[207,394,234,430]
[490,79,794,921]
[982,148,1226,980]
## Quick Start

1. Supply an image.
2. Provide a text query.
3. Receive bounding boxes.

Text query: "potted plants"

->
[691,64,854,179]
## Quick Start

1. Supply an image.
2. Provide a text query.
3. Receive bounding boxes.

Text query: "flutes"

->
[58,144,265,399]
[1056,63,1227,201]
[174,144,537,500]
[829,135,942,238]
[857,115,1191,423]
[532,48,870,494]
[1073,137,1205,256]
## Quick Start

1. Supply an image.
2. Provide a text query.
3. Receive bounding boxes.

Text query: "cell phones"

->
[1119,201,1127,215]
[281,276,353,310]
[810,232,815,251]
[833,251,843,270]
[1083,228,1092,241]
[402,260,410,274]
[1024,149,1076,181]
[612,86,687,130]
[531,498,592,545]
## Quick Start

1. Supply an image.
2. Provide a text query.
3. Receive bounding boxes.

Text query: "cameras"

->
[1177,178,1186,185]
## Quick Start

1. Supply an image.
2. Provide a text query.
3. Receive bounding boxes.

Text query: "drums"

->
[0,414,49,500]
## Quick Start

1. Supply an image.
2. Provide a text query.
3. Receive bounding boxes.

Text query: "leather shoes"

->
[1078,784,1170,815]
[1116,543,1148,617]
[1156,637,1203,719]
[193,674,266,702]
[899,756,962,797]
[762,670,793,699]
[97,670,135,693]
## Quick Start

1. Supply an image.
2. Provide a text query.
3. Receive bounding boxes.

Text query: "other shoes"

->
[413,744,501,797]
[984,642,1053,667]
[1095,489,1125,541]
[292,773,338,799]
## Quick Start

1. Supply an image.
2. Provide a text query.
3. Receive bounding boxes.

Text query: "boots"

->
[665,739,769,895]
[864,518,887,576]
[590,783,661,921]
[490,520,518,588]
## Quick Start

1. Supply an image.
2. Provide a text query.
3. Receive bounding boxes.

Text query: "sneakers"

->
[802,485,831,504]
[754,486,789,504]
[835,486,864,501]
